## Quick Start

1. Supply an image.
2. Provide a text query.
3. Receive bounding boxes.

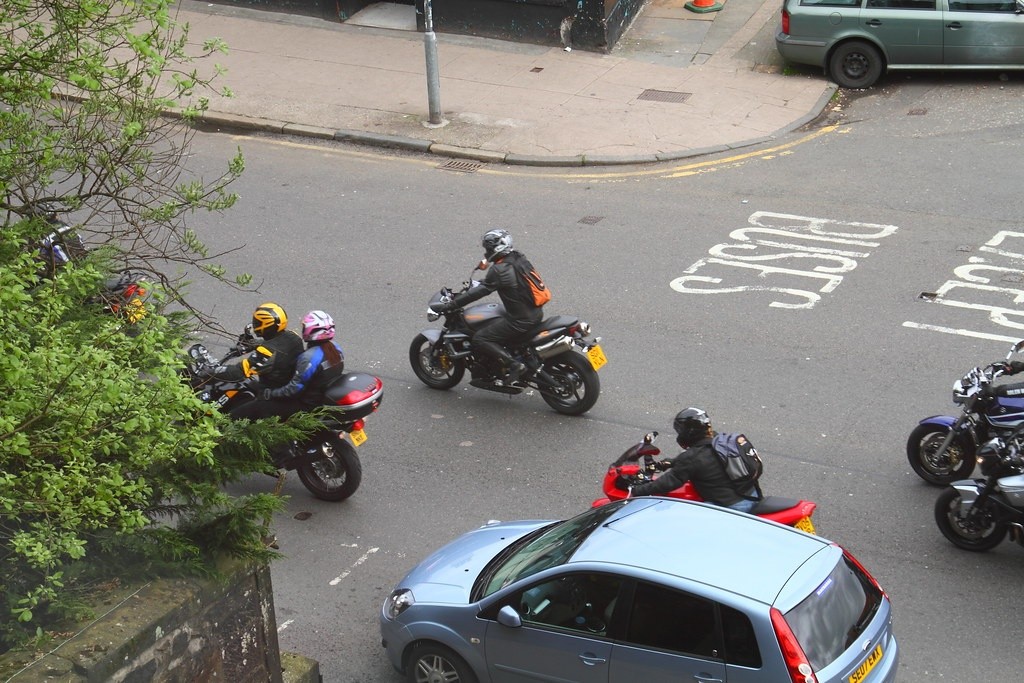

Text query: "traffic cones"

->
[685,0,722,14]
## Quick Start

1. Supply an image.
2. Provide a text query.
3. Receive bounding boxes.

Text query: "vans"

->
[777,0,1024,88]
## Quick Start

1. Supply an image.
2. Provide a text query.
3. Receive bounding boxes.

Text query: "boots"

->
[481,341,528,387]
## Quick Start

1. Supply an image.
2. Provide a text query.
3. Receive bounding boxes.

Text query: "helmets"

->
[253,302,288,338]
[28,201,59,225]
[673,407,712,449]
[481,228,514,262]
[301,310,335,342]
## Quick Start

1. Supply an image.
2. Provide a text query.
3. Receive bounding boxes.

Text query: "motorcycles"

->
[907,340,1024,548]
[594,431,817,550]
[409,259,610,416]
[23,202,147,327]
[161,324,384,502]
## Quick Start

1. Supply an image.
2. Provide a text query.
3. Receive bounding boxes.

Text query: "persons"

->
[433,227,543,388]
[625,406,759,512]
[27,201,86,283]
[203,303,345,470]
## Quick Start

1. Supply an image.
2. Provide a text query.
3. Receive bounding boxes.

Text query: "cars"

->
[379,496,901,683]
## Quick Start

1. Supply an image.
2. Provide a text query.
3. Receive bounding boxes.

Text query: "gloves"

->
[1009,360,1024,376]
[980,384,1007,400]
[656,458,671,472]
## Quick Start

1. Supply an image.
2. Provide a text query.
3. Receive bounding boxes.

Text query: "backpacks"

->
[497,249,551,309]
[695,431,764,502]
